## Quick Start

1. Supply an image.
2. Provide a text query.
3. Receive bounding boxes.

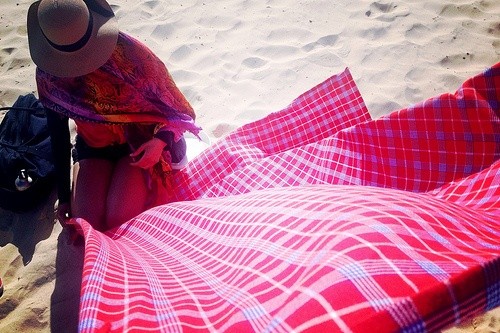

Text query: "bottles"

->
[15,170,32,191]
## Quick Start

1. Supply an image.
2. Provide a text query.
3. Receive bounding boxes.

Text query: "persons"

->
[26,0,201,232]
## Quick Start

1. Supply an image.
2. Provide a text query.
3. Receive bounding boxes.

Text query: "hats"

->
[27,0,119,78]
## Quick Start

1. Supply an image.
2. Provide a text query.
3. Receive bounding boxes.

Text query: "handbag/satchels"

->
[0,94,54,215]
